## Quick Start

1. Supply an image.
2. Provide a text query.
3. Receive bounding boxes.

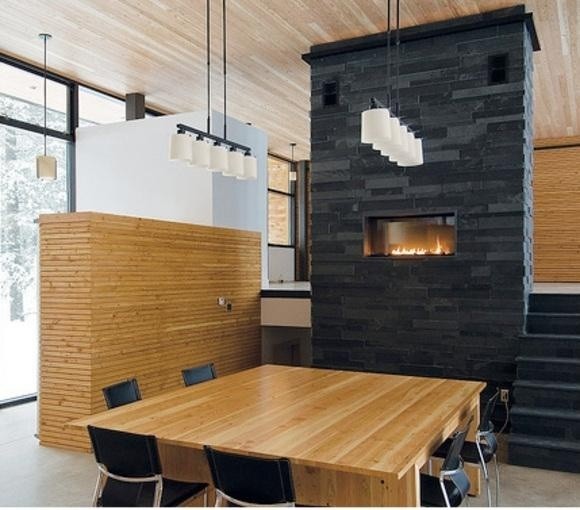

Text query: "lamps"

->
[360,0,424,168]
[36,32,57,183]
[290,143,297,180]
[170,0,259,181]
